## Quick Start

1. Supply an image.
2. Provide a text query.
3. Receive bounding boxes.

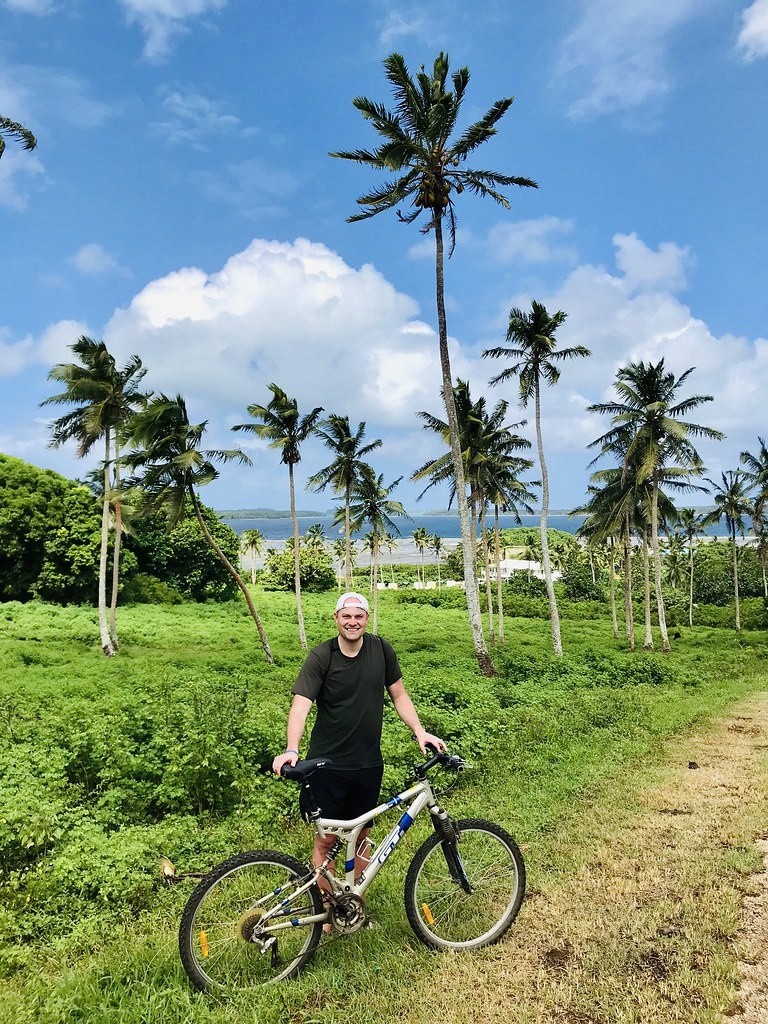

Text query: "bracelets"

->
[286,750,298,755]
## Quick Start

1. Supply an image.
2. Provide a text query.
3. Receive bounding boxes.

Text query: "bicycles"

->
[177,732,527,1002]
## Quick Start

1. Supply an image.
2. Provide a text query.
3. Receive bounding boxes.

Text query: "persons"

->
[273,592,448,933]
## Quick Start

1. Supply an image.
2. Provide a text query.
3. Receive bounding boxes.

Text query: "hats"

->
[334,591,369,614]
